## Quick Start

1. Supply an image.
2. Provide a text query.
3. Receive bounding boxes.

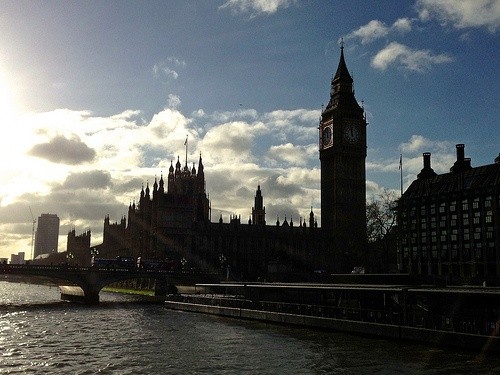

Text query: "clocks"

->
[342,124,359,143]
[321,124,334,150]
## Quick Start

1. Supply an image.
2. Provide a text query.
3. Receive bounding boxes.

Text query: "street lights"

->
[181,256,187,272]
[219,253,230,281]
[65,250,74,267]
[90,248,99,267]
[392,225,403,271]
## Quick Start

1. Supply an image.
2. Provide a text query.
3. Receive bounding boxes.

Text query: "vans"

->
[112,256,134,267]
